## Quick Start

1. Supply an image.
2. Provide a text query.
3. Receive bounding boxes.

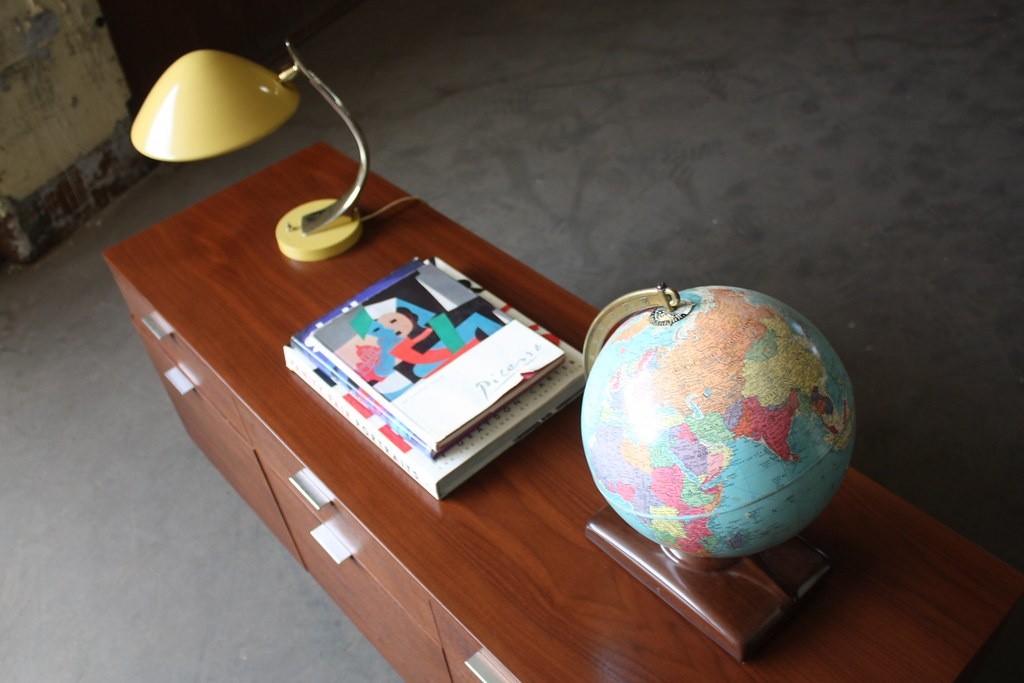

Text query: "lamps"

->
[129,38,369,261]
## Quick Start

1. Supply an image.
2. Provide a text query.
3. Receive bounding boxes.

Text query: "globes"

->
[580,283,864,662]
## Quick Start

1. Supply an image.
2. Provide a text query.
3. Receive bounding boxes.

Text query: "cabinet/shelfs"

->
[103,140,1024,683]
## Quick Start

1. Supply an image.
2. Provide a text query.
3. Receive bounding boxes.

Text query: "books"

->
[280,255,588,498]
